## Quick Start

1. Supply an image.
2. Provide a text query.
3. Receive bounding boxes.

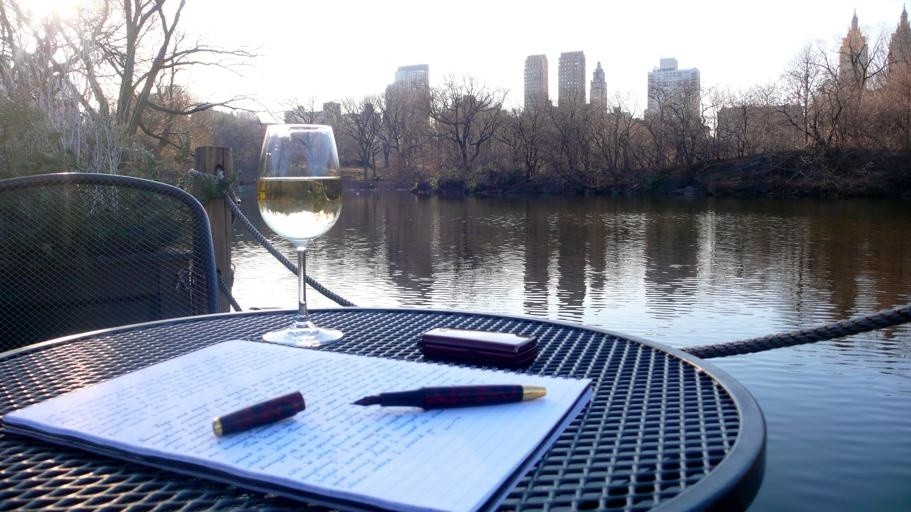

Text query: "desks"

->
[0,305,770,511]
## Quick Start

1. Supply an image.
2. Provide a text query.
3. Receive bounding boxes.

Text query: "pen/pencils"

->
[349,385,547,410]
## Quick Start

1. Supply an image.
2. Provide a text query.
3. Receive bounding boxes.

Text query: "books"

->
[2,336,598,512]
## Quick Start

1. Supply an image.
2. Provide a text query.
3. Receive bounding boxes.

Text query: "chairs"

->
[1,170,218,352]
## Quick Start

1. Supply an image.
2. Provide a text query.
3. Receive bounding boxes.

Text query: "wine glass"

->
[256,123,347,348]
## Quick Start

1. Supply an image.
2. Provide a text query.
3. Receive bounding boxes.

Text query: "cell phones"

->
[418,327,539,360]
